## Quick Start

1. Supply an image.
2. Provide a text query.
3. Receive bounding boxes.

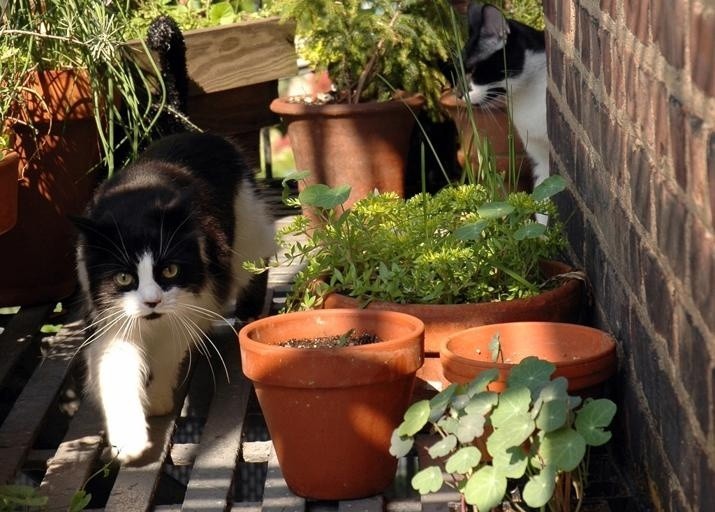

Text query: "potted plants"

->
[238,309,427,500]
[240,0,588,437]
[1,0,167,234]
[439,317,614,495]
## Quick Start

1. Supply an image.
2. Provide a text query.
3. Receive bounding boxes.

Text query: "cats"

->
[49,12,280,466]
[451,0,550,229]
